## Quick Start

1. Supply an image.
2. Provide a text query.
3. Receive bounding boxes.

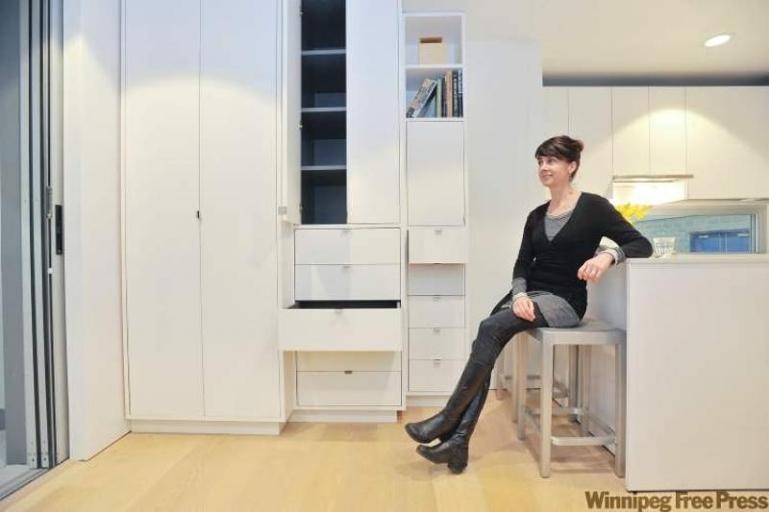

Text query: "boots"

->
[405,353,492,443]
[416,367,491,474]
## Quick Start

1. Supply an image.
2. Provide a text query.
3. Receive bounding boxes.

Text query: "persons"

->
[402,136,654,475]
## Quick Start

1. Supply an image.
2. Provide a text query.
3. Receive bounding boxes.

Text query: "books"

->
[405,69,463,118]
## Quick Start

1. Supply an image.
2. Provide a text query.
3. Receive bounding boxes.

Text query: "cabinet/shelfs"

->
[282,227,409,427]
[119,0,296,435]
[404,13,477,412]
[287,0,405,224]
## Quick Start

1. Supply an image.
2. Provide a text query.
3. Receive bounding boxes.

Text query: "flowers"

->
[610,203,652,224]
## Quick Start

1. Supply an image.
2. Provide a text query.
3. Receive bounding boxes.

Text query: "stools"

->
[494,311,625,481]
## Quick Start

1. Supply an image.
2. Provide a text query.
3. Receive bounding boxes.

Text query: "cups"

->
[651,236,676,262]
